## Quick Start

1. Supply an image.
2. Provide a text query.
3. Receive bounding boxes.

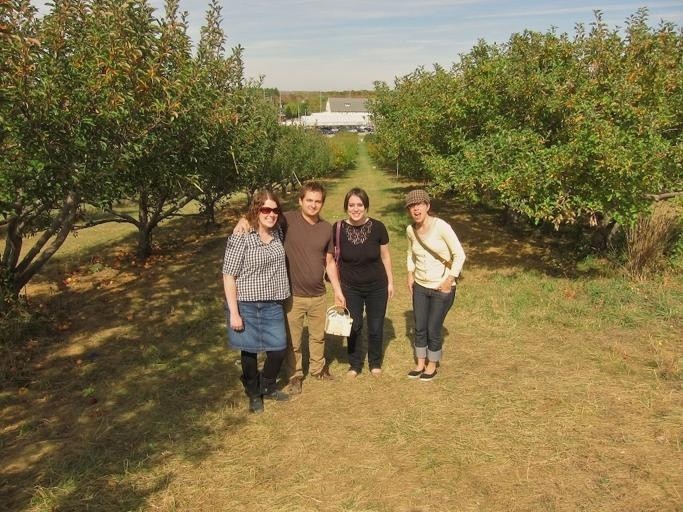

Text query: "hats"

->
[404,190,430,208]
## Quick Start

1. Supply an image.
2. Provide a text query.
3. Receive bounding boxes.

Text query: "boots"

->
[240,370,264,414]
[259,372,289,401]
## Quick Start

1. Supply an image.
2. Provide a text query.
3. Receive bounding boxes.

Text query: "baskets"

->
[325,304,354,337]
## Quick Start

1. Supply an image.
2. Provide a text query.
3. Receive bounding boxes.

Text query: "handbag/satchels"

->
[449,257,465,284]
[325,246,342,282]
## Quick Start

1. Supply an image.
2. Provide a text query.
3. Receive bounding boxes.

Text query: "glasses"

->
[258,207,280,215]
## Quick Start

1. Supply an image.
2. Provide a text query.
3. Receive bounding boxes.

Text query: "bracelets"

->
[447,277,454,282]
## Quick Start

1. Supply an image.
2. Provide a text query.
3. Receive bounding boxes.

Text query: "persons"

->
[222,191,288,415]
[403,190,465,381]
[232,183,346,395]
[333,187,394,379]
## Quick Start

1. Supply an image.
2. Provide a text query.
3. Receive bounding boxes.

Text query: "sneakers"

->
[288,365,437,395]
